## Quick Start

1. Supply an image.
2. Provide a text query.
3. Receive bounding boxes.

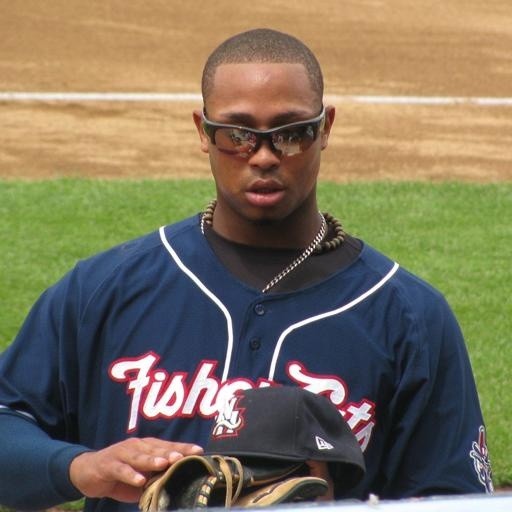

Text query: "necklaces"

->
[200,209,326,295]
[205,196,346,256]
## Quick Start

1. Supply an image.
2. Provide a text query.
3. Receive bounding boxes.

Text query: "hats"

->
[202,387,366,496]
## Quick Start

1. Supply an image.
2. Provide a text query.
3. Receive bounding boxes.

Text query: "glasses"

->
[200,106,325,157]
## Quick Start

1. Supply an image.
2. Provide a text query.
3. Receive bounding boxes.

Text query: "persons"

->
[0,29,493,512]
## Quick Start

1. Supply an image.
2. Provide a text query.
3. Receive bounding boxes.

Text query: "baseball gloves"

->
[138,455,329,508]
